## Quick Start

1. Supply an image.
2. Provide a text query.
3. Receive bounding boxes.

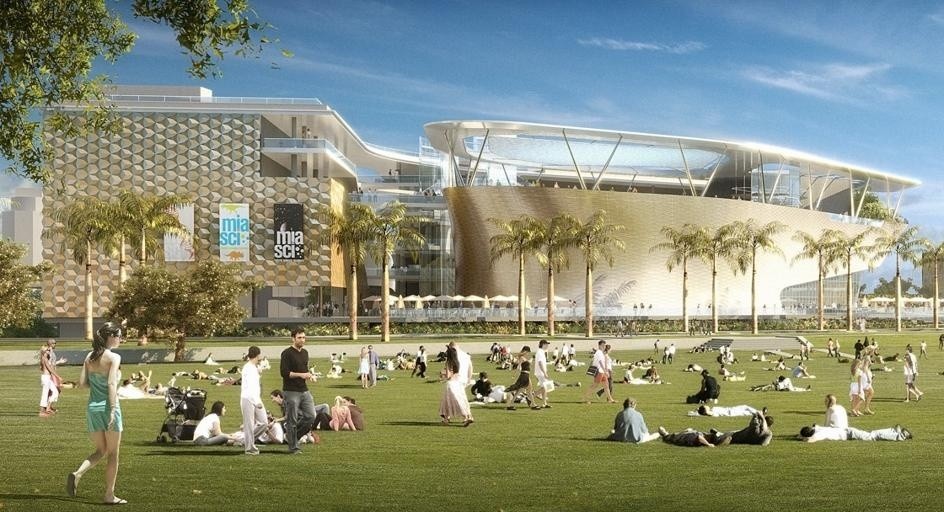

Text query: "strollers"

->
[156,387,208,443]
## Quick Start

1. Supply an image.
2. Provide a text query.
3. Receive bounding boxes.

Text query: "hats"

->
[539,340,550,345]
[523,346,531,352]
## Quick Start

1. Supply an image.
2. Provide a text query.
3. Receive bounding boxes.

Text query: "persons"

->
[582,315,944,447]
[40,338,68,416]
[205,352,219,365]
[242,352,250,360]
[281,329,315,455]
[125,369,191,397]
[257,357,271,374]
[307,302,333,317]
[191,366,243,386]
[270,389,315,444]
[310,339,580,433]
[59,381,79,389]
[193,401,237,447]
[225,409,285,444]
[66,322,129,506]
[240,346,269,456]
[312,395,364,431]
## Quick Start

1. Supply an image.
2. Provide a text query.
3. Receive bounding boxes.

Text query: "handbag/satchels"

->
[586,365,597,376]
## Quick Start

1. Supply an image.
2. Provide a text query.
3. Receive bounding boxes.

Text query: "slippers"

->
[507,406,516,410]
[541,405,552,408]
[526,397,531,407]
[106,498,127,505]
[532,406,540,410]
[67,473,78,498]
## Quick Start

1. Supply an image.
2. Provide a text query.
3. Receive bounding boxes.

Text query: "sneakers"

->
[657,426,669,437]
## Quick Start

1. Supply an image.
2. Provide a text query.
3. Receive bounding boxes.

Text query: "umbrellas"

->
[360,292,576,320]
